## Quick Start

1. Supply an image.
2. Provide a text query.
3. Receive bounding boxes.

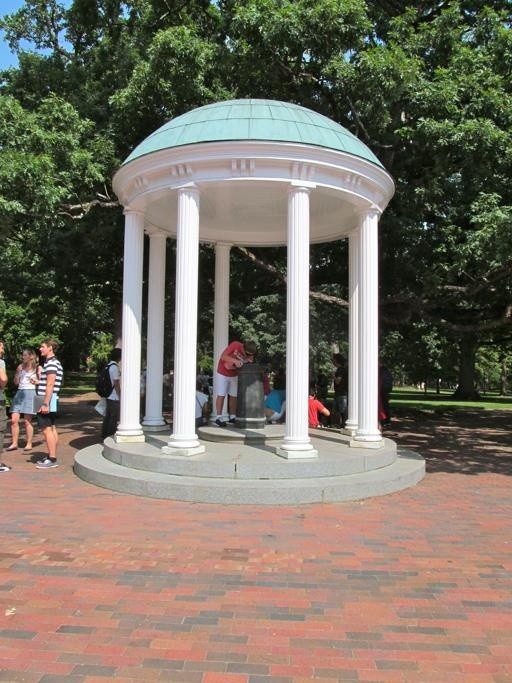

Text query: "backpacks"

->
[94,364,120,397]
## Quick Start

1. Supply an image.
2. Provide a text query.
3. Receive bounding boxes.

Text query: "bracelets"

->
[42,402,49,407]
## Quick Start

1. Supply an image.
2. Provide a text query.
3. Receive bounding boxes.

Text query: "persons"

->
[1,339,14,472]
[30,337,64,468]
[98,339,390,441]
[6,346,43,451]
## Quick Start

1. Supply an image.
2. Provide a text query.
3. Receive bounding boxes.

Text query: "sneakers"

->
[23,447,33,451]
[0,463,12,473]
[36,453,59,468]
[214,416,240,427]
[5,445,18,451]
